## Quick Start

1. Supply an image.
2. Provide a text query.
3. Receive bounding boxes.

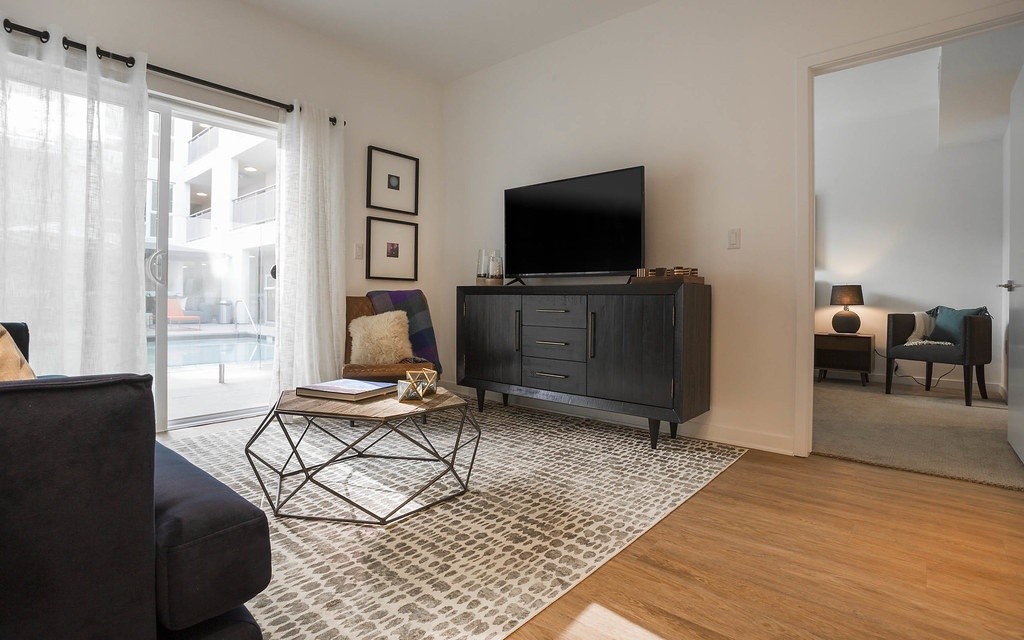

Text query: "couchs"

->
[0,322,271,640]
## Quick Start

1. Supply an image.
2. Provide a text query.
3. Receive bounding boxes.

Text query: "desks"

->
[244,386,481,525]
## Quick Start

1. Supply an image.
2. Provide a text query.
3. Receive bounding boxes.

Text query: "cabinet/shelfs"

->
[456,284,712,449]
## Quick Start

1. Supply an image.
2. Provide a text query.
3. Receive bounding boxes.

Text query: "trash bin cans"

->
[218,300,233,324]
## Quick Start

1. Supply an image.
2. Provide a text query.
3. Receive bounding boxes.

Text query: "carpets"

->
[811,378,1024,492]
[158,388,750,640]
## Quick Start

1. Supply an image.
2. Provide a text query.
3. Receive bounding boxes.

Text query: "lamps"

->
[830,285,865,333]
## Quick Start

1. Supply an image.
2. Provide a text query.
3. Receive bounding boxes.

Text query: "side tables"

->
[814,332,875,387]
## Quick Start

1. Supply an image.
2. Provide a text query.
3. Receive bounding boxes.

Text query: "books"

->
[296,378,397,402]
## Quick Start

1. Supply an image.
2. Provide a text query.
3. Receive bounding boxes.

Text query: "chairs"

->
[167,299,201,332]
[342,296,441,427]
[886,314,992,406]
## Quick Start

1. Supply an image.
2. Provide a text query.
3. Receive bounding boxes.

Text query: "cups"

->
[477,248,499,277]
[488,256,503,279]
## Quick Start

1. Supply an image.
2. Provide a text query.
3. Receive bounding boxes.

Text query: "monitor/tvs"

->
[505,165,646,286]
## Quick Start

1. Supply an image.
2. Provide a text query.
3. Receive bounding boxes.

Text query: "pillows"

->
[348,310,413,366]
[928,305,987,348]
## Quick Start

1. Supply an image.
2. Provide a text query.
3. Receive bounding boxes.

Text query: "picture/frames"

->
[366,216,418,281]
[366,145,420,215]
